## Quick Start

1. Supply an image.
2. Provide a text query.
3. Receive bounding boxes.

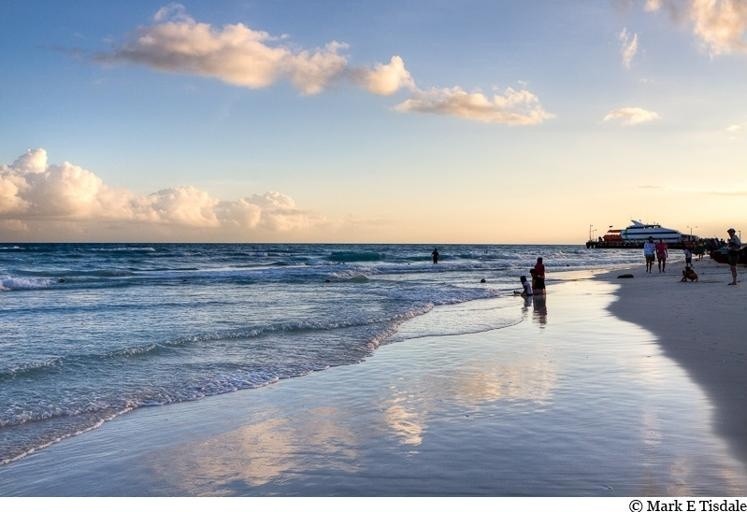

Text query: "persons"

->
[654,238,667,272]
[641,234,656,274]
[726,228,741,286]
[431,248,439,264]
[677,233,728,282]
[532,257,544,292]
[511,274,531,296]
[530,269,535,277]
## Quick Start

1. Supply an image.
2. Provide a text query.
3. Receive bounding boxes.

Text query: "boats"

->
[584,218,702,255]
